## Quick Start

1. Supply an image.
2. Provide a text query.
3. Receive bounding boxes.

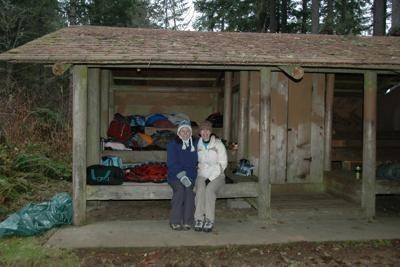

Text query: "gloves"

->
[204,178,211,187]
[176,170,192,188]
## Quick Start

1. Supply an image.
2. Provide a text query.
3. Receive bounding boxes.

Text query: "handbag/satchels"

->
[236,158,255,177]
[85,163,124,186]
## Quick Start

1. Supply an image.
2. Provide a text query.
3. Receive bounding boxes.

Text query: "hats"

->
[177,119,192,142]
[199,120,213,132]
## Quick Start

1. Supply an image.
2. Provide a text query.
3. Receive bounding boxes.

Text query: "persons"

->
[165,119,202,230]
[194,120,229,232]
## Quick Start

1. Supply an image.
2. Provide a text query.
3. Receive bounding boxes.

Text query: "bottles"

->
[355,166,360,180]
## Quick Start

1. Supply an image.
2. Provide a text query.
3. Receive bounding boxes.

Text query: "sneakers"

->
[169,223,192,232]
[194,219,214,233]
